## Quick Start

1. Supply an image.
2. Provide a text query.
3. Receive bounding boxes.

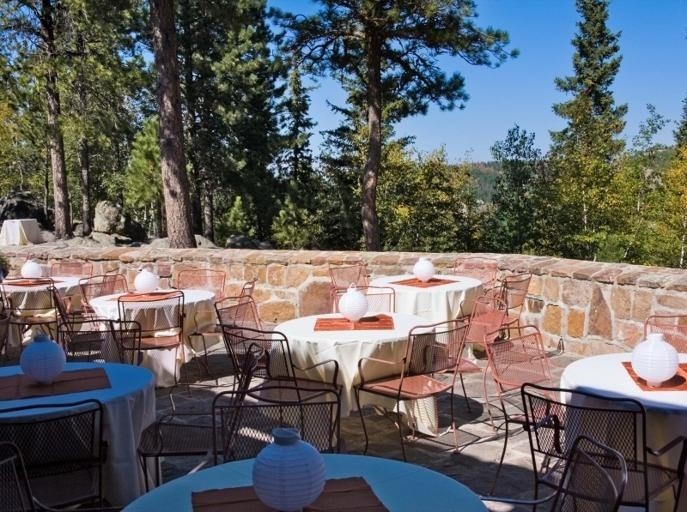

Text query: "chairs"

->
[136,343,265,493]
[79,274,129,310]
[50,261,94,277]
[57,319,141,366]
[435,296,508,438]
[47,285,88,322]
[453,256,498,293]
[484,324,565,497]
[0,441,125,512]
[458,273,531,341]
[520,382,687,512]
[330,264,370,288]
[188,277,258,375]
[212,384,341,466]
[0,398,109,508]
[105,267,120,275]
[354,316,470,464]
[213,294,270,405]
[119,291,191,392]
[217,324,344,453]
[1,277,62,356]
[480,435,628,512]
[643,315,687,354]
[330,285,395,314]
[177,268,227,375]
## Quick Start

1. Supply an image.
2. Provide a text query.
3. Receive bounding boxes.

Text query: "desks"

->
[120,453,490,512]
[367,273,483,361]
[89,289,216,375]
[559,352,687,512]
[273,313,436,418]
[0,363,157,505]
[0,276,88,345]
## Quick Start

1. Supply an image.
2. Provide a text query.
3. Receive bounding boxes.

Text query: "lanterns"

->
[339,282,368,322]
[631,333,679,387]
[135,269,159,293]
[252,427,325,512]
[413,257,434,282]
[20,334,66,386]
[21,260,42,278]
